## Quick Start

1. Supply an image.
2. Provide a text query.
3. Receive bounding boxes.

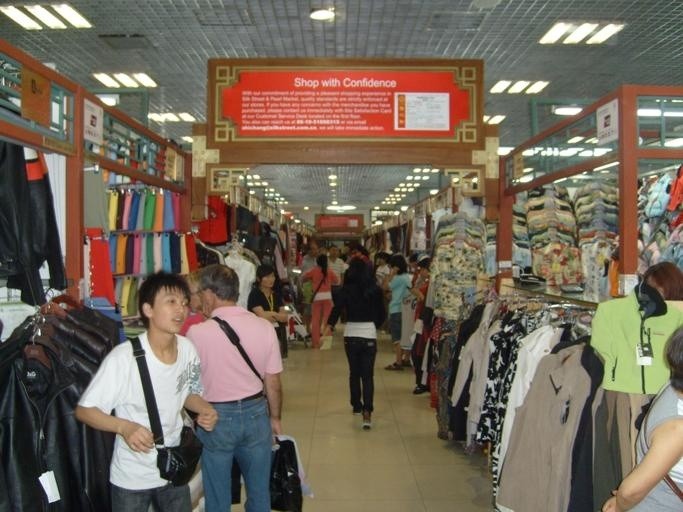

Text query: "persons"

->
[248,263,291,360]
[76,270,217,510]
[641,260,682,301]
[316,252,386,434]
[599,323,682,512]
[406,257,433,395]
[299,235,419,378]
[183,260,283,511]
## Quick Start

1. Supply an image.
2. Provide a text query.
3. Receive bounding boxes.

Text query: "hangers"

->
[431,171,621,261]
[478,288,593,344]
[17,281,122,395]
[637,168,681,273]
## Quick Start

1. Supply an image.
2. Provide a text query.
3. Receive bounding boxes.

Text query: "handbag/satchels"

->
[157,427,203,487]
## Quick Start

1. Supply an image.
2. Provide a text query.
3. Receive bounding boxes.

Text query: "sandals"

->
[384,362,402,370]
